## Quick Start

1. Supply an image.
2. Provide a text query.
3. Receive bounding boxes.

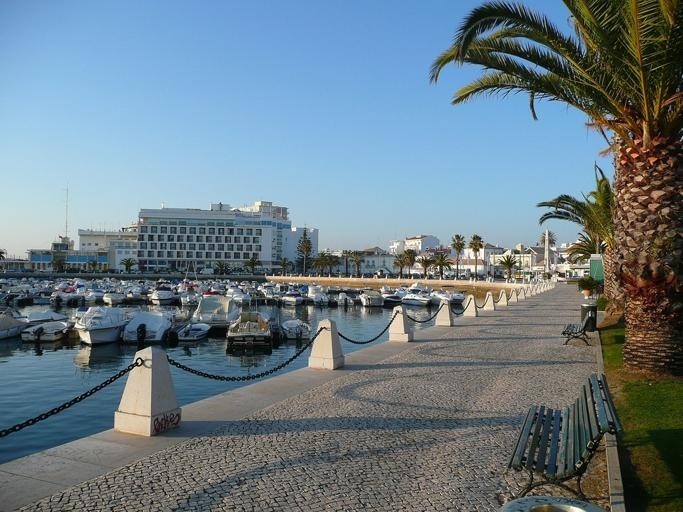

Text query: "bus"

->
[443,268,471,279]
[513,270,534,280]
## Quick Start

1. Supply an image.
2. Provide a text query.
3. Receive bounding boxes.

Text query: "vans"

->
[199,268,213,275]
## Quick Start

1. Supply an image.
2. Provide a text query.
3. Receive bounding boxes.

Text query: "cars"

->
[9,267,124,274]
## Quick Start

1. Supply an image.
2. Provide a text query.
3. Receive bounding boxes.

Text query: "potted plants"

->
[577,276,599,296]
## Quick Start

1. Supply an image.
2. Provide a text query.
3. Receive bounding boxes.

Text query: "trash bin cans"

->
[581,303,597,331]
[500,495,606,512]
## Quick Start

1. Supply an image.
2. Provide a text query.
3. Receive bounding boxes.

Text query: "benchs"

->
[506,371,623,503]
[560,310,595,346]
